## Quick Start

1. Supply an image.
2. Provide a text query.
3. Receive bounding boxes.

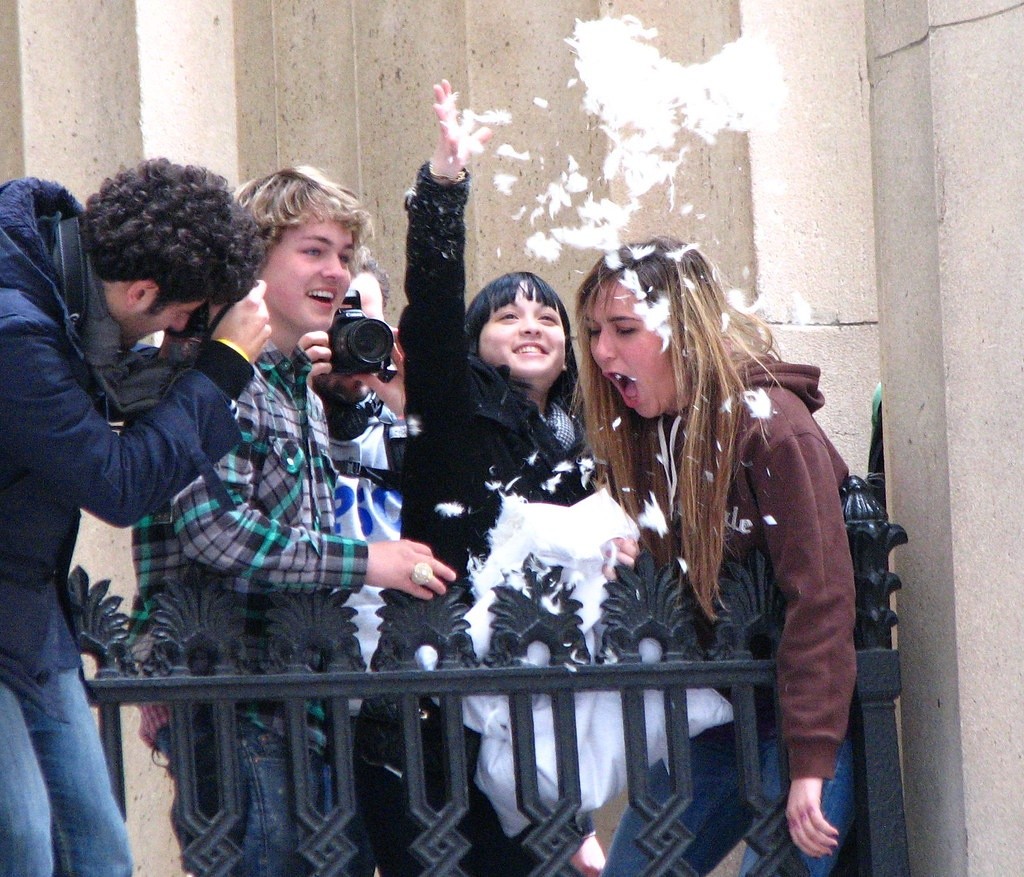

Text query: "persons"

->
[131,164,404,877]
[358,78,595,877]
[0,155,273,877]
[577,234,858,877]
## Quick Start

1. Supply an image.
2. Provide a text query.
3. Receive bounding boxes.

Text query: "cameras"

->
[170,276,249,338]
[318,290,394,376]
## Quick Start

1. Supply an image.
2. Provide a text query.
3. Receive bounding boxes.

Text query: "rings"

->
[409,561,434,586]
